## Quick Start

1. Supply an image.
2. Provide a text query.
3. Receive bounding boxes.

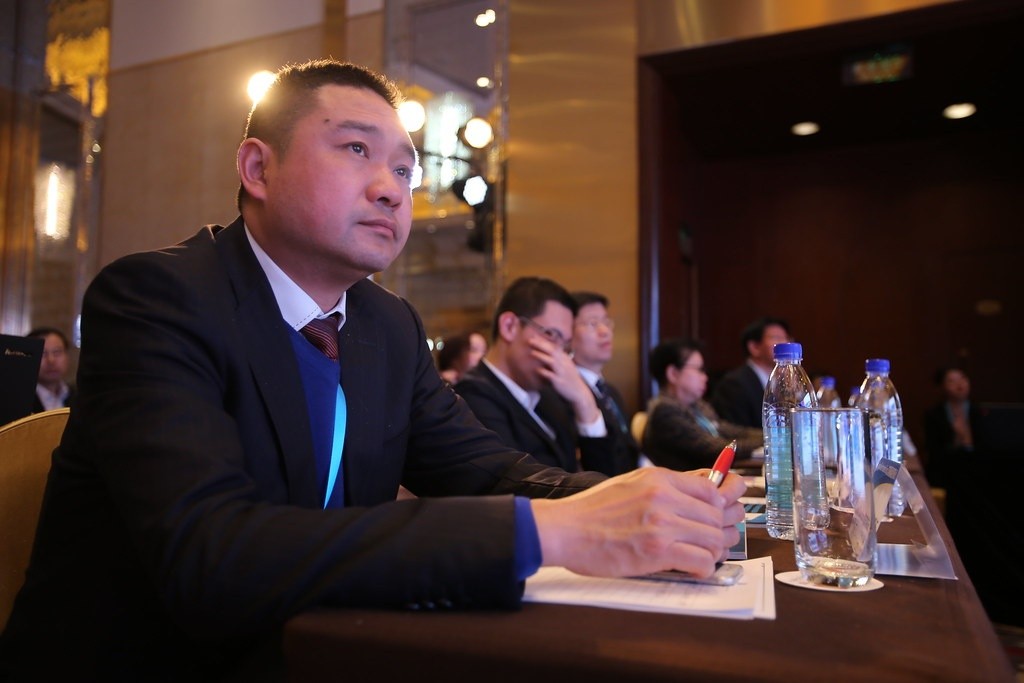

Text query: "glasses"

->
[575,319,616,332]
[518,316,575,358]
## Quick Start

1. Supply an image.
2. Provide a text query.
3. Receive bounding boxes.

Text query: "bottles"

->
[762,343,829,541]
[817,377,841,410]
[853,356,906,518]
[848,388,861,408]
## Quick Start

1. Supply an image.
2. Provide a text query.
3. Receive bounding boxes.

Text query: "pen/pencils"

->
[706,439,741,491]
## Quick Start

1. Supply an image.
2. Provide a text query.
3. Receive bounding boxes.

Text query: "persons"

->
[638,340,765,472]
[0,56,747,683]
[570,291,633,441]
[437,331,487,383]
[712,314,794,430]
[924,363,1024,627]
[23,327,74,429]
[449,276,641,479]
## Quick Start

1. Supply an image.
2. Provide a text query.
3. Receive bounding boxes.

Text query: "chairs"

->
[0,407,71,632]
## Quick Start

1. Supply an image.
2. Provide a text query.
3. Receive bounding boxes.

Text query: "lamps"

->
[457,116,494,154]
[450,174,495,212]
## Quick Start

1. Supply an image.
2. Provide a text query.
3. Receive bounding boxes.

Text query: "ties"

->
[952,406,971,446]
[595,379,627,432]
[300,312,341,360]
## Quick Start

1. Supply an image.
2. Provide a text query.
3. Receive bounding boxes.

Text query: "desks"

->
[269,449,1024,683]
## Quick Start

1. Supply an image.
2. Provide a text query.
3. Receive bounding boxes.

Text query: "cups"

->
[790,407,877,588]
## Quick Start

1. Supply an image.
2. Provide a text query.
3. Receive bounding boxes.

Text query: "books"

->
[731,494,767,528]
[709,518,747,561]
